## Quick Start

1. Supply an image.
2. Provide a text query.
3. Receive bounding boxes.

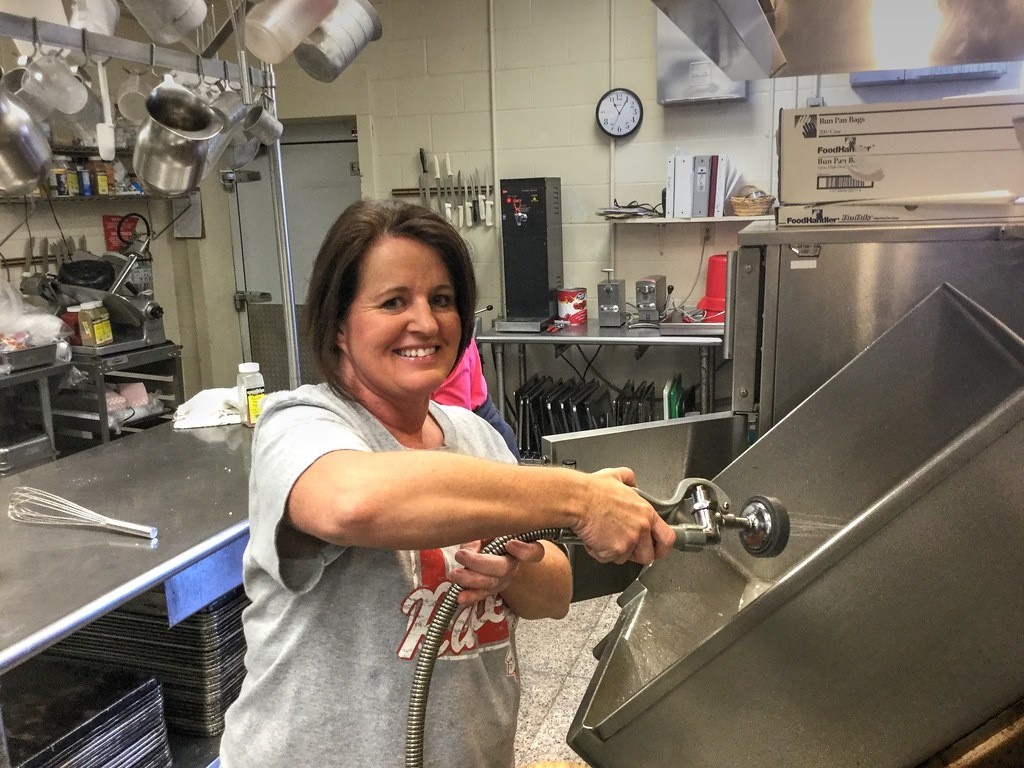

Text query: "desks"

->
[0,363,74,457]
[475,318,722,465]
[0,420,256,680]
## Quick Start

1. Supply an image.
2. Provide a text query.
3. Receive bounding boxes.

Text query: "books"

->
[665,156,675,219]
[673,156,695,219]
[714,154,729,217]
[692,155,712,217]
[708,156,718,216]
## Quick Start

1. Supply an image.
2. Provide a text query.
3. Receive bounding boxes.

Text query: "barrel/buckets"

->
[1,0,385,203]
[556,287,587,325]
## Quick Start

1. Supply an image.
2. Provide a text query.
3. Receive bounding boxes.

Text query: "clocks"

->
[595,88,643,138]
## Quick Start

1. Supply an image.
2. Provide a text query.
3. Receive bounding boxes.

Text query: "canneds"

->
[45,153,118,196]
[236,359,268,429]
[77,300,116,347]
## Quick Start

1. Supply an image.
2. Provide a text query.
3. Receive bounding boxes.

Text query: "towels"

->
[172,384,247,429]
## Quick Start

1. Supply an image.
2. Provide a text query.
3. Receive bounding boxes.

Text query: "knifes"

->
[417,147,494,228]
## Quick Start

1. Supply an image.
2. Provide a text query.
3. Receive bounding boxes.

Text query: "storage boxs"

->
[728,195,775,215]
[565,279,1022,768]
[774,92,1023,230]
[739,185,766,198]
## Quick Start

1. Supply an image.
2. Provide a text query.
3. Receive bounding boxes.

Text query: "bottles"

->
[89,300,114,345]
[235,361,267,430]
[76,303,107,349]
[61,306,82,348]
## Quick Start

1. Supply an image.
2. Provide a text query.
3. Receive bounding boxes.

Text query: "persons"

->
[216,195,679,768]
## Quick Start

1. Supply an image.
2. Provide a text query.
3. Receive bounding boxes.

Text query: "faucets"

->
[617,474,793,559]
[511,198,527,226]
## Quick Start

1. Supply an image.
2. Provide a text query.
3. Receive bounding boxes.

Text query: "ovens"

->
[713,214,1023,448]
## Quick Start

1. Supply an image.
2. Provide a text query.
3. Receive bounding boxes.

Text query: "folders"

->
[665,153,728,218]
